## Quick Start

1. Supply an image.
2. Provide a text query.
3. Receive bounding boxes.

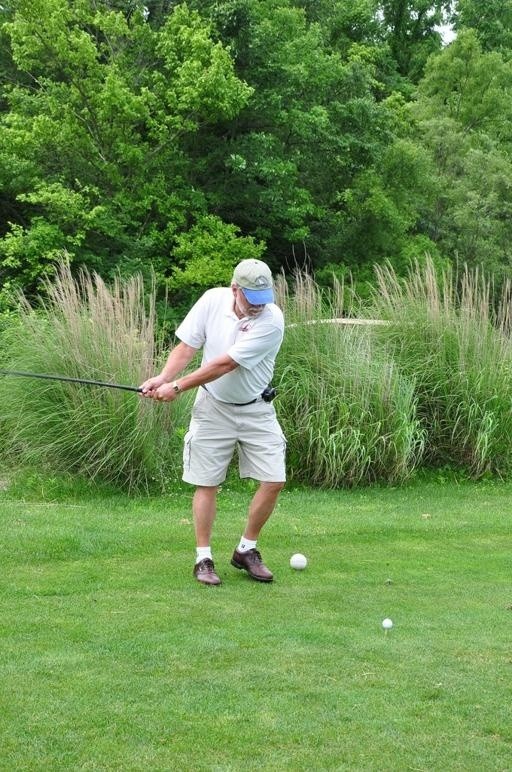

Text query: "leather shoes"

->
[195,558,222,585]
[230,546,274,581]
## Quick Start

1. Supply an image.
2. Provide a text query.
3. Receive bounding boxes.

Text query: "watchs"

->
[171,379,182,395]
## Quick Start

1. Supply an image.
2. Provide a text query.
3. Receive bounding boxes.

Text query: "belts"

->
[202,384,257,406]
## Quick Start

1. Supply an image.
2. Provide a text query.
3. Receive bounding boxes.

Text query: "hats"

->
[234,258,275,305]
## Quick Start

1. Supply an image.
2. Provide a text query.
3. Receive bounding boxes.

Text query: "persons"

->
[137,258,288,586]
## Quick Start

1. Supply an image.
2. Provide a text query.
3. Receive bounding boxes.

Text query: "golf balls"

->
[291,553,307,570]
[383,618,393,628]
